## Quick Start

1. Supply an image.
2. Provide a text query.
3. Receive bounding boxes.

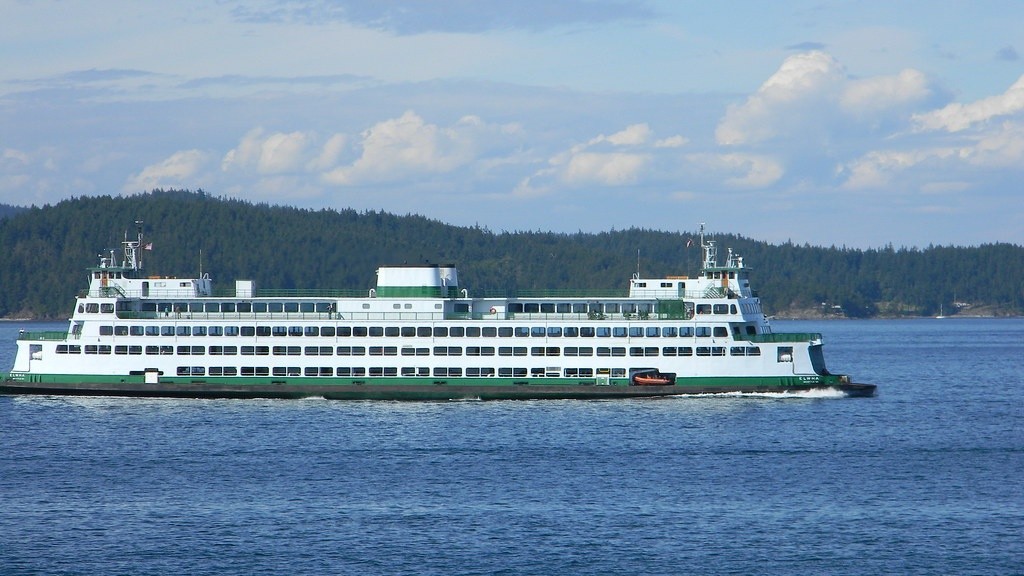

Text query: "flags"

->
[144,242,152,250]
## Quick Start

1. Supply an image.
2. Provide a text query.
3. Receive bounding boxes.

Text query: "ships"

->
[0,219,877,400]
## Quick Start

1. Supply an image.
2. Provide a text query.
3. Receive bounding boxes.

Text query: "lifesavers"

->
[490,308,496,314]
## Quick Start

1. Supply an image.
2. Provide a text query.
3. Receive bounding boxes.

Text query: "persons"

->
[165,306,168,318]
[176,304,181,319]
